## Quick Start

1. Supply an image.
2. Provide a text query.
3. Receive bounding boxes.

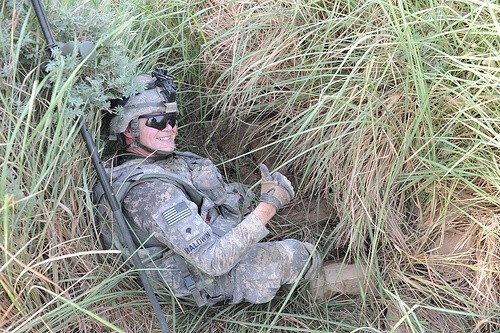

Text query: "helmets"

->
[106,72,179,139]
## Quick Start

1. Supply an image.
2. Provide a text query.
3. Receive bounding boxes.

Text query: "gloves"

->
[257,163,295,211]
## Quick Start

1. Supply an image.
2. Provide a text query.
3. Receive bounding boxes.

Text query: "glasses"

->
[137,114,175,130]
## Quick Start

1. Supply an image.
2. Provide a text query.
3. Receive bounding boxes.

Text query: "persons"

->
[94,71,375,305]
